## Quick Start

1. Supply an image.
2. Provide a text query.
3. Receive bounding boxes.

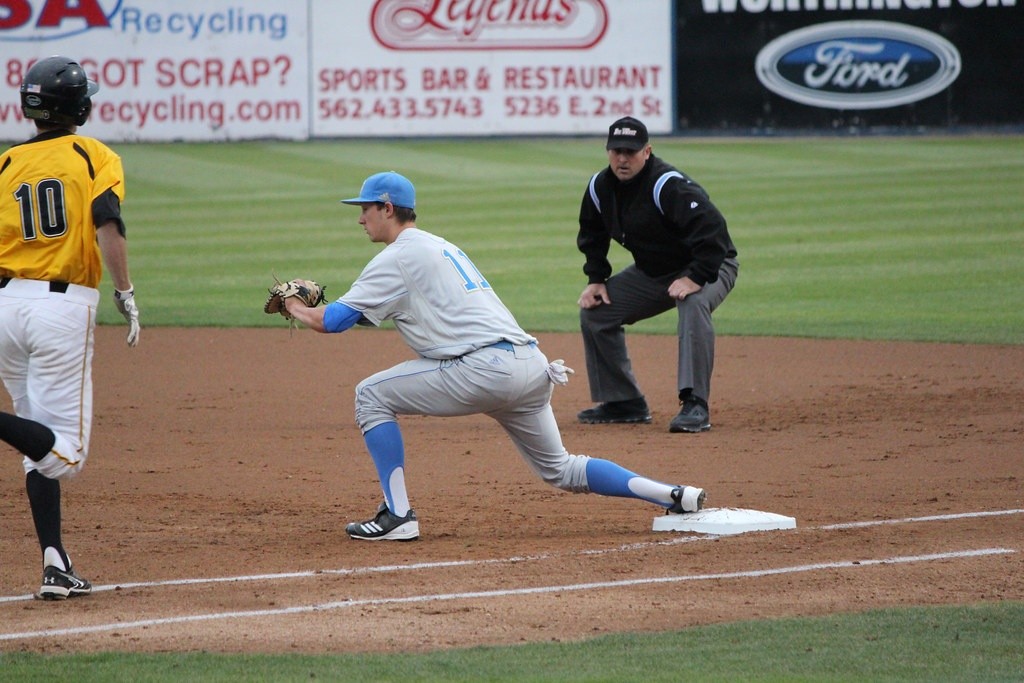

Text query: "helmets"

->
[18,53,98,123]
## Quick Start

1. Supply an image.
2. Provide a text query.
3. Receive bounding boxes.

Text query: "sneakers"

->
[344,501,422,540]
[671,397,714,434]
[665,481,707,515]
[39,561,95,599]
[577,399,652,423]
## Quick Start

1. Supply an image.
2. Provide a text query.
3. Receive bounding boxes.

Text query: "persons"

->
[0,56,141,601]
[264,170,706,541]
[576,116,740,433]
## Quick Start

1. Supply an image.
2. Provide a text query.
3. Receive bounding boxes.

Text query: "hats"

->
[338,167,417,211]
[606,116,649,153]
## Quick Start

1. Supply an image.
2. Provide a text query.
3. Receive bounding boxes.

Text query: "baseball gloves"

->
[264,271,328,339]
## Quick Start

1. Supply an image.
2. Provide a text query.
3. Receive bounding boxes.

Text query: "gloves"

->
[113,285,141,348]
